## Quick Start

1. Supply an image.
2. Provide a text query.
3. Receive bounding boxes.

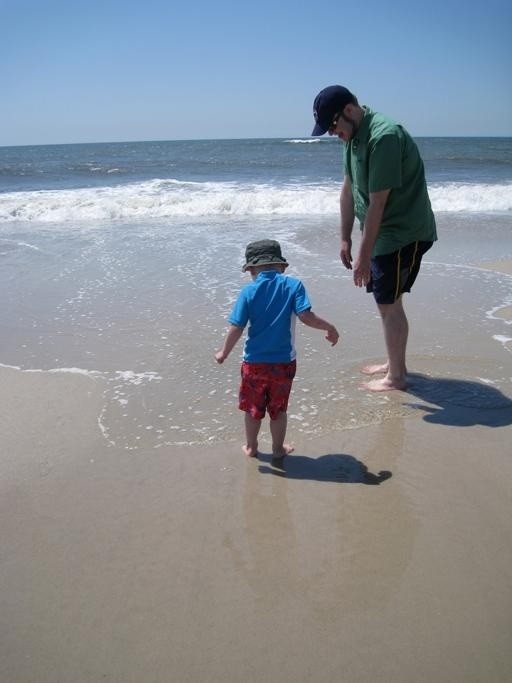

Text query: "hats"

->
[242,240,289,273]
[311,85,352,136]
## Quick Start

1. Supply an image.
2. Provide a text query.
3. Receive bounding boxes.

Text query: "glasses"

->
[329,113,342,130]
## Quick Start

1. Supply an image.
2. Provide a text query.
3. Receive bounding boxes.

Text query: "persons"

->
[213,238,340,459]
[310,85,438,393]
